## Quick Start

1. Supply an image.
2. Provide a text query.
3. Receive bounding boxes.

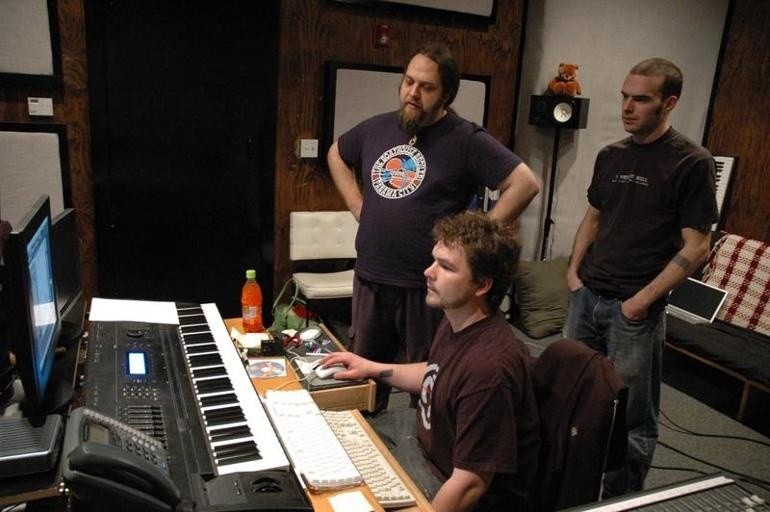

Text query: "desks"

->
[222,317,436,511]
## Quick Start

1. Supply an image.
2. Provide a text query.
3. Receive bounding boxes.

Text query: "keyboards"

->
[321,408,418,507]
[262,388,364,492]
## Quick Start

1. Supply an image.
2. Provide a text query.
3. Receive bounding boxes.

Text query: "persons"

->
[326,41,541,416]
[562,54,724,506]
[314,209,547,511]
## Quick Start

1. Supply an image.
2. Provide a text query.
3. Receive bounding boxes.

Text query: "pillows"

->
[515,257,570,337]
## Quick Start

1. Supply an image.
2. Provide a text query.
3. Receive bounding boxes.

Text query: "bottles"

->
[240,270,264,332]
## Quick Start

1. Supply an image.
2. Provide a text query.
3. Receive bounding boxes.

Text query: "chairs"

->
[288,210,360,326]
[534,340,629,511]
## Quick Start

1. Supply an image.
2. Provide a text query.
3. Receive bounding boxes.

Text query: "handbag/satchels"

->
[267,304,324,333]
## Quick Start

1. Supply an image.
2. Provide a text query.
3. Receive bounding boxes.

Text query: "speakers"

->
[528,92,592,130]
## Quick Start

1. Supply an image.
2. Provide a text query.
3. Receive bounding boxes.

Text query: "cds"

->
[247,359,286,378]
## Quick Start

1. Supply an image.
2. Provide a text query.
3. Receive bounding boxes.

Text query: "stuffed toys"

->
[544,62,584,98]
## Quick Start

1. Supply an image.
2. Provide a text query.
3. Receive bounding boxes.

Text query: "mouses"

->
[314,361,349,380]
[300,324,323,344]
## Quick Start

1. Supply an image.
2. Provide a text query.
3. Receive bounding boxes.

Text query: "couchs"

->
[667,232,770,420]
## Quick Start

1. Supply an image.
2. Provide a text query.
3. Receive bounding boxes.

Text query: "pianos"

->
[85,298,316,511]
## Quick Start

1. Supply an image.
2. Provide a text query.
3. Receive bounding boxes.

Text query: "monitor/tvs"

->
[51,205,87,340]
[6,191,76,428]
